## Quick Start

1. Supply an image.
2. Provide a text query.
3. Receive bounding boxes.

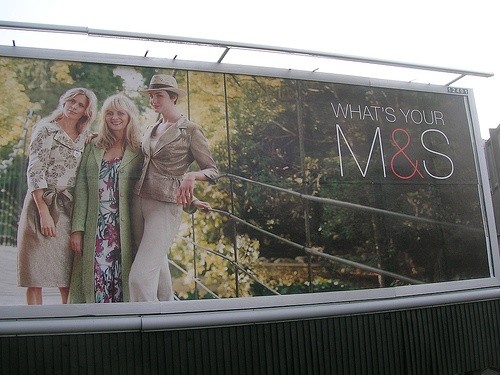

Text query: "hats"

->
[139,74,185,99]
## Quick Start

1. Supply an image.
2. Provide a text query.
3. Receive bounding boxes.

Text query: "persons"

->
[16,87,98,306]
[65,95,213,304]
[83,72,220,303]
